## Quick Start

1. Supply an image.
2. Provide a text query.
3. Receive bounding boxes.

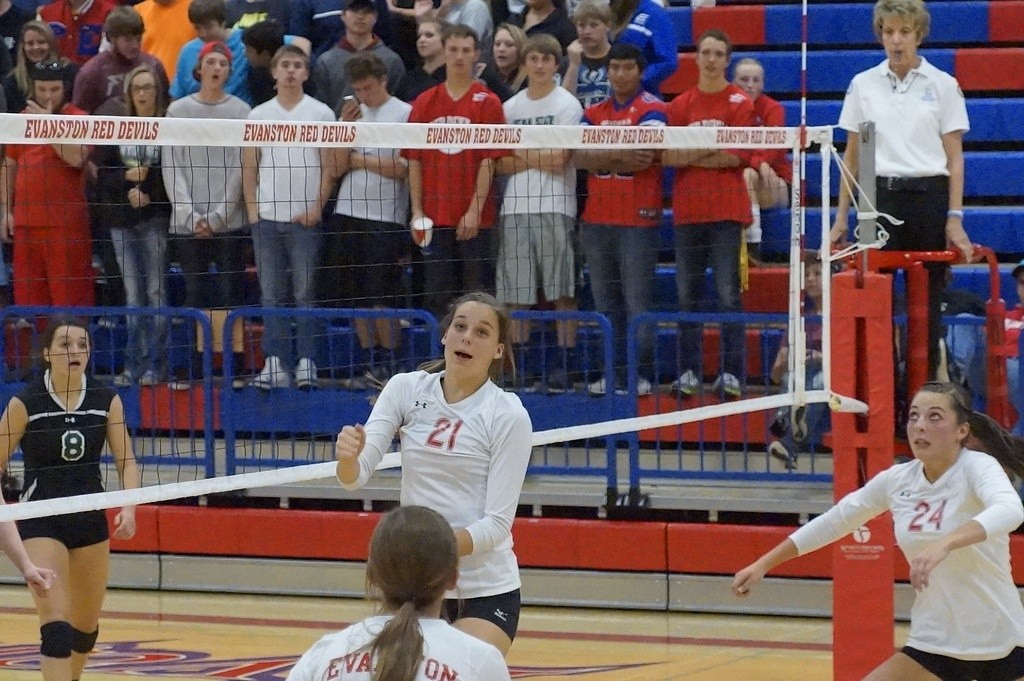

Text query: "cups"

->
[410,217,433,248]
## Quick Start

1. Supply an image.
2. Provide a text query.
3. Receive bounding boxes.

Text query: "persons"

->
[575,44,665,396]
[241,46,336,393]
[731,56,807,242]
[817,0,976,438]
[333,292,533,660]
[763,249,842,471]
[730,380,1024,681]
[0,490,56,598]
[0,58,96,387]
[398,23,513,393]
[96,64,172,386]
[494,32,584,393]
[0,2,792,401]
[660,28,761,399]
[327,54,413,390]
[279,505,512,681]
[0,314,141,681]
[159,41,253,390]
[936,258,1024,400]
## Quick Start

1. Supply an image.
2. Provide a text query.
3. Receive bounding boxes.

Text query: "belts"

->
[877,177,937,192]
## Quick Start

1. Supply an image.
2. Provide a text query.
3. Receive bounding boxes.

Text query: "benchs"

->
[0,1,1024,442]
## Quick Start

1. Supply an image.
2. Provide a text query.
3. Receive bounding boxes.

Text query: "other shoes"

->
[672,368,700,394]
[113,370,132,387]
[233,356,289,389]
[505,379,541,393]
[295,358,319,388]
[588,378,607,394]
[615,377,653,396]
[771,438,799,469]
[138,369,160,385]
[718,372,742,396]
[549,377,575,394]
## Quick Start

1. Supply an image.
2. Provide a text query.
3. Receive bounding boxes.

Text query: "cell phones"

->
[343,94,363,119]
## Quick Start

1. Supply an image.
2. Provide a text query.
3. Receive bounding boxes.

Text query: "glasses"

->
[35,63,63,71]
[130,84,157,90]
[350,5,377,13]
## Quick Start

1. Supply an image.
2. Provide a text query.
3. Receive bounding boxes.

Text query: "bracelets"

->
[946,209,965,219]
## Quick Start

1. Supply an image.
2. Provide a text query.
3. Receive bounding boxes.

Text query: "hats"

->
[33,62,64,80]
[1012,259,1024,278]
[193,41,231,81]
[342,0,379,10]
